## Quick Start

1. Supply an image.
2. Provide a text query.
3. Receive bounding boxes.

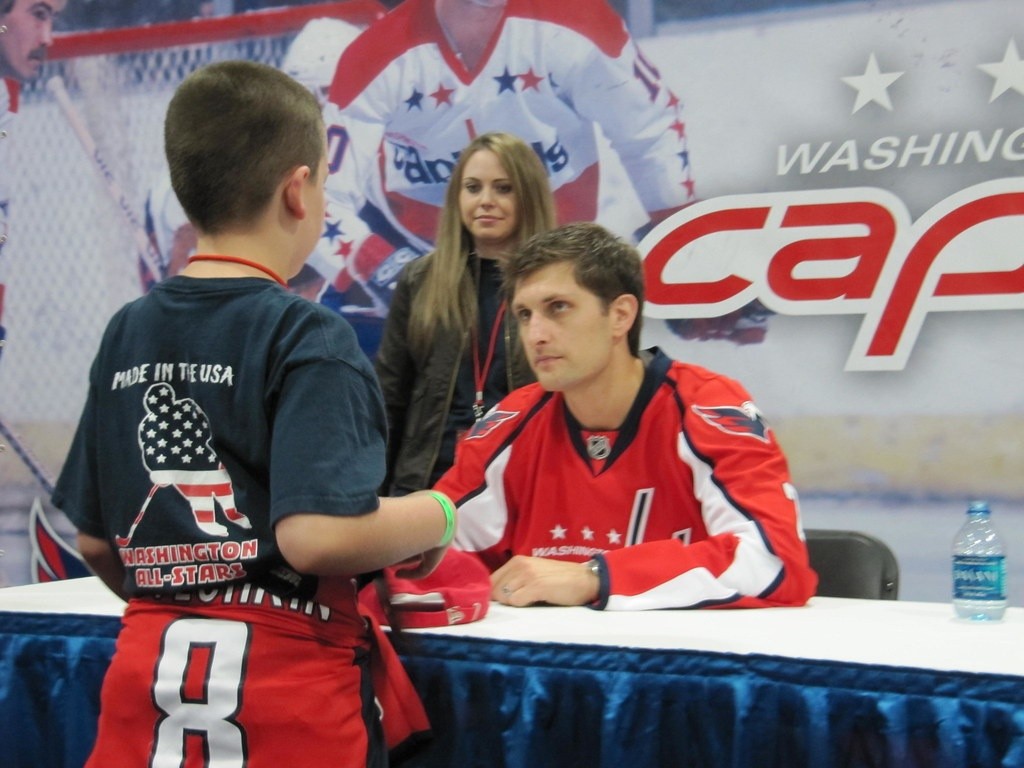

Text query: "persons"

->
[430,223,818,612]
[372,132,560,497]
[0,0,774,373]
[48,61,456,768]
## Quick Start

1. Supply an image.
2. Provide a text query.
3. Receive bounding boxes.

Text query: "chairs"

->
[804,527,899,600]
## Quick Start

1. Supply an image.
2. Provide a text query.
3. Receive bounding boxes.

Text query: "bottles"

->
[950,498,1010,619]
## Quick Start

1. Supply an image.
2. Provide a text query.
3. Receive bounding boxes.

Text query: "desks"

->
[0,575,1024,768]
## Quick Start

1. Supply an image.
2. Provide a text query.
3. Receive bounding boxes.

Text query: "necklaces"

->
[187,253,289,288]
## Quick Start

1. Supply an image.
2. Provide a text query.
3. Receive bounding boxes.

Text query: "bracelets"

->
[415,489,458,547]
[584,558,602,574]
